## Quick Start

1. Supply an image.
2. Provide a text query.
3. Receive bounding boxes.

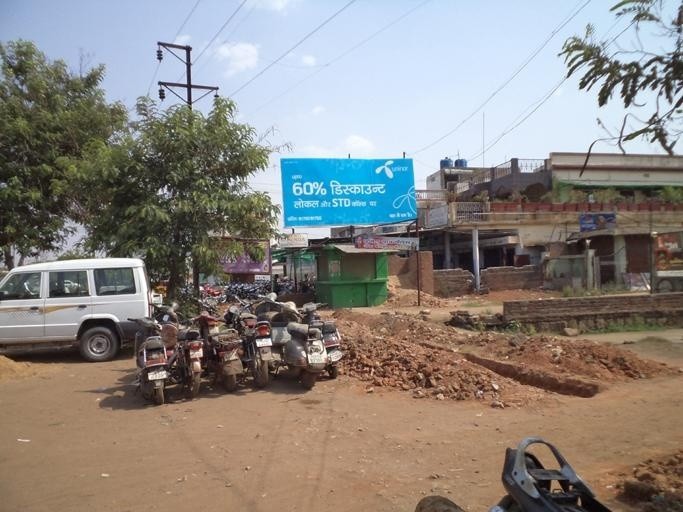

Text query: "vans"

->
[0,254,154,363]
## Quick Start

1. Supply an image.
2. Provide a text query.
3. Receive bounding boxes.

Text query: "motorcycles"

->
[487,435,612,510]
[126,273,346,407]
